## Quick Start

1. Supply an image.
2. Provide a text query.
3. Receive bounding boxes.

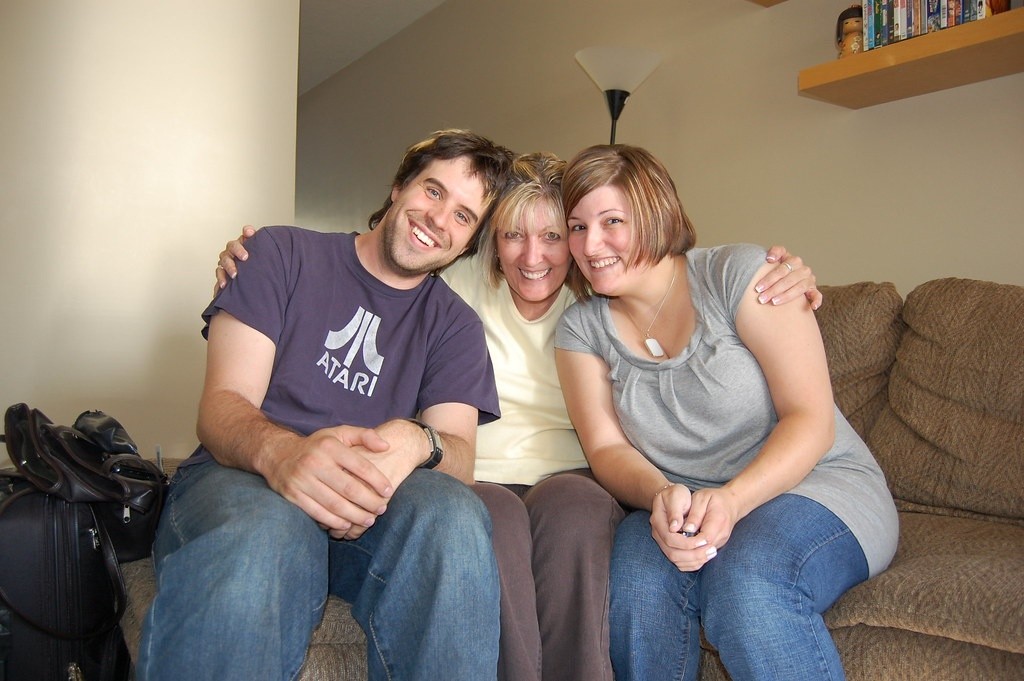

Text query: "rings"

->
[217,265,224,270]
[783,261,793,272]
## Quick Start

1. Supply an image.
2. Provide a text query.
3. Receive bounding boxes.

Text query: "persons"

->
[551,143,899,681]
[212,152,823,681]
[134,128,514,681]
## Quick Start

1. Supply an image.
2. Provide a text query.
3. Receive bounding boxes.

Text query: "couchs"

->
[119,280,1024,681]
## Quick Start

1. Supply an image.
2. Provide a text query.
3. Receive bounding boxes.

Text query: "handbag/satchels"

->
[0,403,171,642]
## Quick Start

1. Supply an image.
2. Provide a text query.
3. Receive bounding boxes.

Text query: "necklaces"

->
[619,254,678,358]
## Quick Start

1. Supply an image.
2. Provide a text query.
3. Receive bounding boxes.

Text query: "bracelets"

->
[655,482,674,497]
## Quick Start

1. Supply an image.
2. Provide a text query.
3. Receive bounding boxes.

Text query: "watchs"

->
[405,418,444,469]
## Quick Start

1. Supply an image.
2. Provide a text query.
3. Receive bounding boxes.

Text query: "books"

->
[862,0,1024,52]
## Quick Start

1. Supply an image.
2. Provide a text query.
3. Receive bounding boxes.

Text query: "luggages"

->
[0,476,132,681]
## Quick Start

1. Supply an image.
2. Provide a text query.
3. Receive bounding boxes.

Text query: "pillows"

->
[804,280,1024,525]
